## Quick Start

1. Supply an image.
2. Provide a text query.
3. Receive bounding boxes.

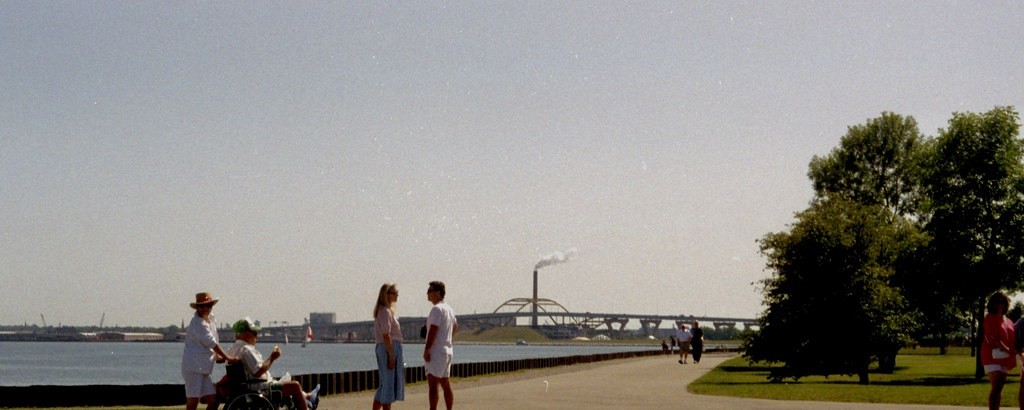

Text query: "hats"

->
[233,316,262,336]
[190,292,219,309]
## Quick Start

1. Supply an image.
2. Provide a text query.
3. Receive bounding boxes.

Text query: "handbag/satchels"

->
[992,347,1010,359]
[420,324,428,339]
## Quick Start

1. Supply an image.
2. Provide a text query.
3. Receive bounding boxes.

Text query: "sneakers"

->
[308,384,320,410]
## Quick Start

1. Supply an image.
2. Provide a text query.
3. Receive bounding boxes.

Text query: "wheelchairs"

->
[214,357,319,410]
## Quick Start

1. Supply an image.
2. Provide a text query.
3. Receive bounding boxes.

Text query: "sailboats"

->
[301,317,312,348]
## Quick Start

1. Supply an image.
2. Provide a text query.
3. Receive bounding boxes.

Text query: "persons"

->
[661,322,704,364]
[182,293,320,410]
[423,281,458,410]
[372,284,404,410]
[981,292,1024,410]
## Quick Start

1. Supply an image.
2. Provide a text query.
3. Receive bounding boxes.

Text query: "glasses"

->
[252,332,257,337]
[389,290,398,295]
[199,304,213,308]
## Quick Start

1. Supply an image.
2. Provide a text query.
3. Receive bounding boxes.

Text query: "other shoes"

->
[678,360,687,365]
